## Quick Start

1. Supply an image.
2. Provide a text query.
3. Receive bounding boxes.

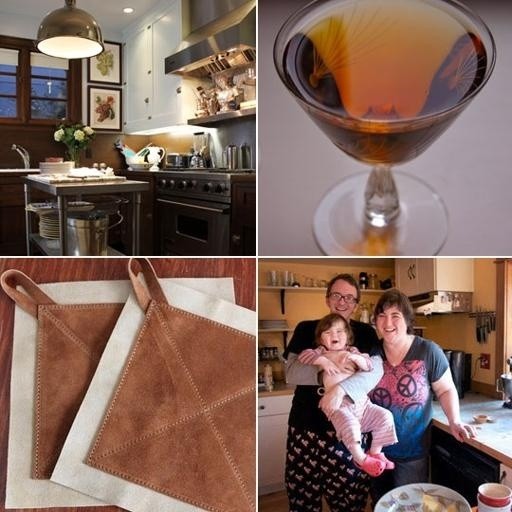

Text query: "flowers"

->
[50,119,98,168]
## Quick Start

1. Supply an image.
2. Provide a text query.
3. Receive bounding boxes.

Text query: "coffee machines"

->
[442,349,472,399]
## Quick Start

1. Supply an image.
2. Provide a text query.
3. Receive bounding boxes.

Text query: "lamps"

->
[33,0,106,63]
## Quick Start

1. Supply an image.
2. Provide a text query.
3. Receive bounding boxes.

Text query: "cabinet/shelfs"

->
[97,171,158,256]
[119,1,208,140]
[258,388,299,499]
[392,258,474,302]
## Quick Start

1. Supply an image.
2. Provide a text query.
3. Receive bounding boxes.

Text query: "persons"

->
[297,312,398,478]
[280,273,385,511]
[367,288,477,512]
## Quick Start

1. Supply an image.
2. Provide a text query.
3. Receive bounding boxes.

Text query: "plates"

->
[38,161,76,177]
[260,316,289,330]
[373,483,473,512]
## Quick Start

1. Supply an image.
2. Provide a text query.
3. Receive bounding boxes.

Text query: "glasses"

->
[327,292,357,303]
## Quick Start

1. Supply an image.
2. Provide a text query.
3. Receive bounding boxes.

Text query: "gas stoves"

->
[155,168,256,196]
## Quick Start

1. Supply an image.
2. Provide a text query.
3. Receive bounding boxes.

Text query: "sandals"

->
[365,448,395,469]
[353,453,386,477]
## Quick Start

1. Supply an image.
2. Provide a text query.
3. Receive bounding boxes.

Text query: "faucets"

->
[11,144,30,169]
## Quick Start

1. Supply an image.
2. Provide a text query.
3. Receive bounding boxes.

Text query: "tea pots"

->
[380,278,392,290]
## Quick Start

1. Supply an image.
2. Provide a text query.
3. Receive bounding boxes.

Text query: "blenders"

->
[189,131,208,169]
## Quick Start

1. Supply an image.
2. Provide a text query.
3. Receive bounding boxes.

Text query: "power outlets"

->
[497,461,511,491]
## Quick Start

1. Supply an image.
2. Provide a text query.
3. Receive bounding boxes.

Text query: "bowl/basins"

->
[273,370,285,383]
[37,213,59,241]
[473,415,497,424]
[125,162,155,173]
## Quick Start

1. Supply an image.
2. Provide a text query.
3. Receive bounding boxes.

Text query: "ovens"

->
[431,441,498,505]
[152,194,232,256]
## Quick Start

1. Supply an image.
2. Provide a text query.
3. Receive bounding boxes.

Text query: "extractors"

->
[407,291,474,316]
[166,1,255,78]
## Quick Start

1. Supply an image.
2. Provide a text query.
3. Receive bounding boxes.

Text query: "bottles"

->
[360,272,377,289]
[93,163,107,170]
[359,300,375,325]
[263,363,275,391]
[260,346,280,359]
[240,142,254,169]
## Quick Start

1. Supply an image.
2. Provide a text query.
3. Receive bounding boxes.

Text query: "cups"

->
[496,373,512,408]
[265,270,327,286]
[477,483,512,512]
[222,143,238,170]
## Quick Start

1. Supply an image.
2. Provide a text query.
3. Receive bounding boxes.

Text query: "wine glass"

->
[272,0,497,255]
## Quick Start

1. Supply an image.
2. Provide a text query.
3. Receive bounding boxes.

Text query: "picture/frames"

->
[87,38,123,86]
[86,84,124,132]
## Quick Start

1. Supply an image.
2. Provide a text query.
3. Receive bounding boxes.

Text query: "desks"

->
[429,399,512,509]
